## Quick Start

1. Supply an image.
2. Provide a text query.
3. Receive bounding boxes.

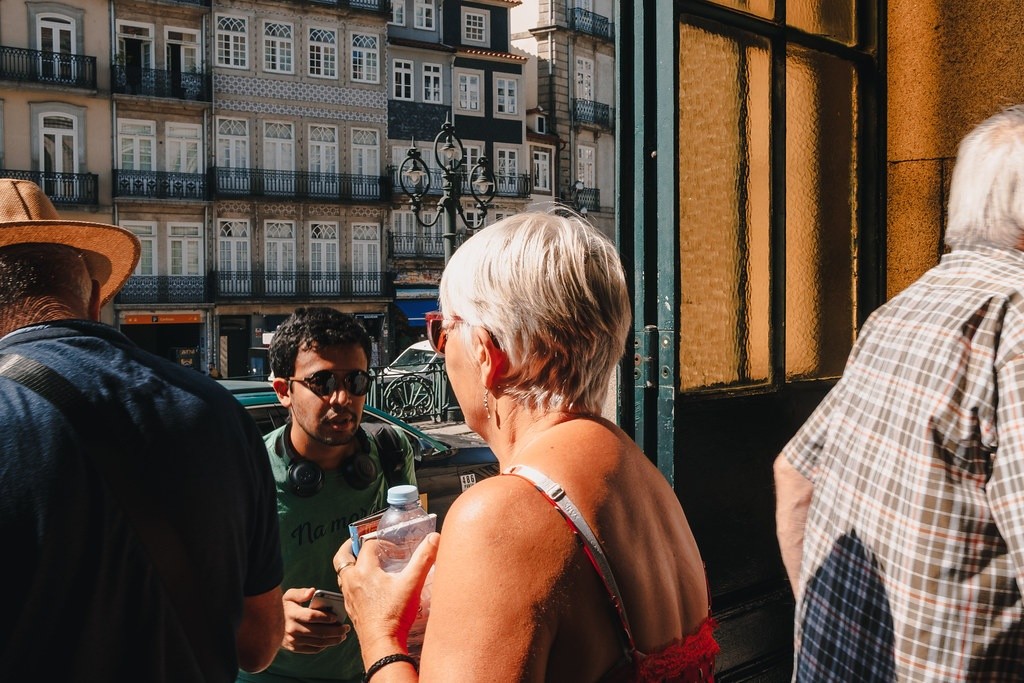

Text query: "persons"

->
[333,200,721,683]
[0,178,288,683]
[235,308,418,683]
[772,104,1023,682]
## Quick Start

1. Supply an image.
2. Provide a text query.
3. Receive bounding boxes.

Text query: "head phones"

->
[282,419,379,495]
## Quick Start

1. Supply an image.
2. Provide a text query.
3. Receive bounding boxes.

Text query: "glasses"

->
[285,369,374,396]
[426,311,468,357]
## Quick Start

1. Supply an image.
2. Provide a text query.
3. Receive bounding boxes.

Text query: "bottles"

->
[376,485,435,677]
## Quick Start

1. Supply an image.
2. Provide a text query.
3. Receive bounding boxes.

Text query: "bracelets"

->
[362,653,418,683]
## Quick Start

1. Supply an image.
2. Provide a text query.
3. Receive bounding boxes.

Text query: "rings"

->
[336,561,355,574]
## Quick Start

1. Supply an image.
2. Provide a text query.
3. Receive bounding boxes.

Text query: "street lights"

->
[397,108,499,262]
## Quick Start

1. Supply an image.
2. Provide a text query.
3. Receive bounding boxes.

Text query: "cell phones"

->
[308,588,349,626]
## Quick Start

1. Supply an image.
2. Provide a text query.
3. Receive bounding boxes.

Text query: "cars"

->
[213,378,289,432]
[377,340,445,399]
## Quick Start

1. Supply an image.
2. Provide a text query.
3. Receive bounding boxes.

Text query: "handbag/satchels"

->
[0,353,238,683]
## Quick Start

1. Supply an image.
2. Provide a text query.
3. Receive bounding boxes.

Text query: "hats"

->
[0,178,142,310]
